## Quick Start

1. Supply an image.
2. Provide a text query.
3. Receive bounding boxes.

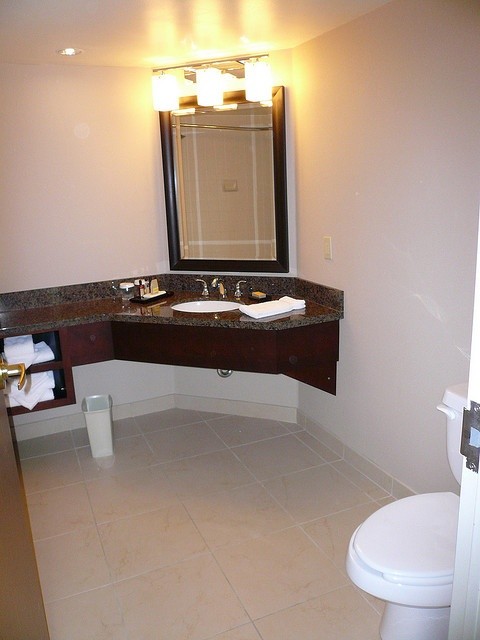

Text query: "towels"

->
[4,391,54,407]
[6,371,52,411]
[4,335,36,364]
[238,296,306,320]
[2,341,56,366]
[48,372,55,389]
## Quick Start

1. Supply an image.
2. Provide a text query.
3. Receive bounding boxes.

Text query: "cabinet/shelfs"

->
[0,319,76,420]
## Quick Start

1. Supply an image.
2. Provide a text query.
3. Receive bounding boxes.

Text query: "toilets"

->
[345,382,467,640]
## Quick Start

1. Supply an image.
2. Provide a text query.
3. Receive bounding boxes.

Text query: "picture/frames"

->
[160,86,290,272]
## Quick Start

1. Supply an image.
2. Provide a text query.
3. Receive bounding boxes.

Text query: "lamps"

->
[245,61,274,103]
[195,66,223,106]
[151,70,180,112]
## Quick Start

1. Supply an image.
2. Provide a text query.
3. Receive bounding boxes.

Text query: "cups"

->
[134,279,149,297]
[119,283,134,300]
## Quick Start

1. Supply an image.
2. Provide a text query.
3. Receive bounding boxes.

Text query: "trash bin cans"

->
[81,394,114,458]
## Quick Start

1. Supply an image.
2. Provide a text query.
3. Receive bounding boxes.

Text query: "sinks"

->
[171,301,240,312]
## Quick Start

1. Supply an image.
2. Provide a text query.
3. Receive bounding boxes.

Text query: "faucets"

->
[216,280,227,299]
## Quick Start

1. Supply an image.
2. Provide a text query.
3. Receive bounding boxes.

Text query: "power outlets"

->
[323,236,332,259]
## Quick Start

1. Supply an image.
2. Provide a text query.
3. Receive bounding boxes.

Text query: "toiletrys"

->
[137,280,145,296]
